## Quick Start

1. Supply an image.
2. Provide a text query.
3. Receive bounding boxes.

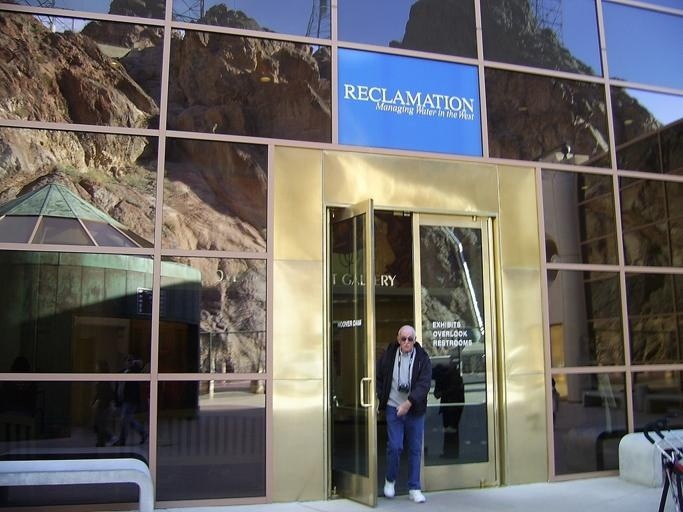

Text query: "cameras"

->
[397,383,410,393]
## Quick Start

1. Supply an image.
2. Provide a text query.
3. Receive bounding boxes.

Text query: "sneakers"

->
[408,490,426,502]
[384,478,396,498]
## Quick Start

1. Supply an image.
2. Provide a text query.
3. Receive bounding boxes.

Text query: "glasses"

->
[401,336,412,341]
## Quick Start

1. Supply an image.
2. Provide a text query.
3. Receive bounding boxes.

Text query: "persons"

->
[429,358,466,459]
[373,323,434,502]
[0,354,37,452]
[109,352,149,449]
[88,359,119,448]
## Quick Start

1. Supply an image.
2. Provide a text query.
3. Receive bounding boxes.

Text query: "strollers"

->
[642,418,682,512]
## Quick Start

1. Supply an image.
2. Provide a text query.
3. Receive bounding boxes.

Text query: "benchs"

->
[0,458,154,512]
[0,446,149,465]
[596,424,683,471]
[618,429,683,486]
